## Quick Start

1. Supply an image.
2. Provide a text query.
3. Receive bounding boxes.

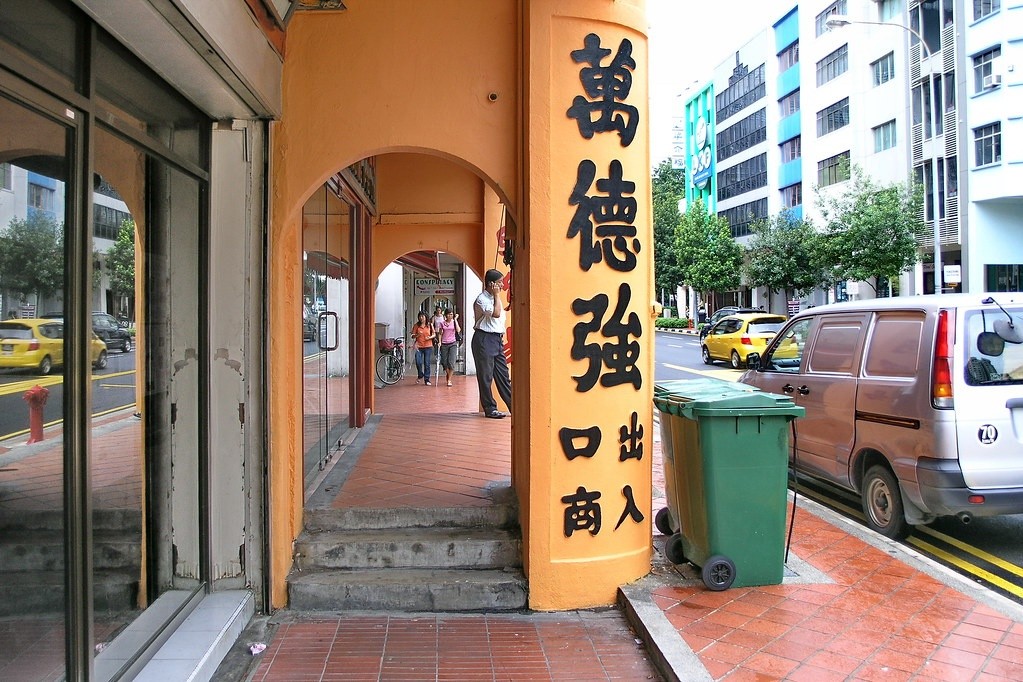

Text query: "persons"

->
[438,307,461,386]
[411,309,436,386]
[472,269,511,419]
[430,306,444,354]
[698,299,705,310]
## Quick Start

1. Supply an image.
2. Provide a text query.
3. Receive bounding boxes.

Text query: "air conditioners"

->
[983,73,1002,88]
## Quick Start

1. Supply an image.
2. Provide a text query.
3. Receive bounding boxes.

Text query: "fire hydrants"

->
[23,386,50,444]
[688,319,692,330]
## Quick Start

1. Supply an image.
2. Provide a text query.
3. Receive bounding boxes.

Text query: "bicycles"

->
[375,336,405,385]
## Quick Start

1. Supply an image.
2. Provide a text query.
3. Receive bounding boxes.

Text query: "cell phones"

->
[489,281,494,287]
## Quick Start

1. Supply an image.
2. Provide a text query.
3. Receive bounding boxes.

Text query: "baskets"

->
[380,339,395,354]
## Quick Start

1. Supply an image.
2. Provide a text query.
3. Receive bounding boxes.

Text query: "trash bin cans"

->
[652,379,807,592]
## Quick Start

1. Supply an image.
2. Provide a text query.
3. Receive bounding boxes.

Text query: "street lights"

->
[826,12,945,294]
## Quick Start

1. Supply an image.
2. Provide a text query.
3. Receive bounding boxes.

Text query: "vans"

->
[312,300,326,321]
[736,293,1023,542]
[303,303,317,342]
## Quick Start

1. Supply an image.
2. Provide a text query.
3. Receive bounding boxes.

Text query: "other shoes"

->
[448,380,452,387]
[415,377,423,384]
[485,410,506,418]
[425,380,431,385]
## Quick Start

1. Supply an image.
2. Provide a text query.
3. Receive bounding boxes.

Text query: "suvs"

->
[91,311,133,352]
[699,306,768,347]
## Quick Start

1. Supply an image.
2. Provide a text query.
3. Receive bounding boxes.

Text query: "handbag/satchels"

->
[430,322,438,346]
[454,321,463,341]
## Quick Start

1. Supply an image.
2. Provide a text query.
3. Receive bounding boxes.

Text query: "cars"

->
[701,313,800,369]
[0,318,108,374]
